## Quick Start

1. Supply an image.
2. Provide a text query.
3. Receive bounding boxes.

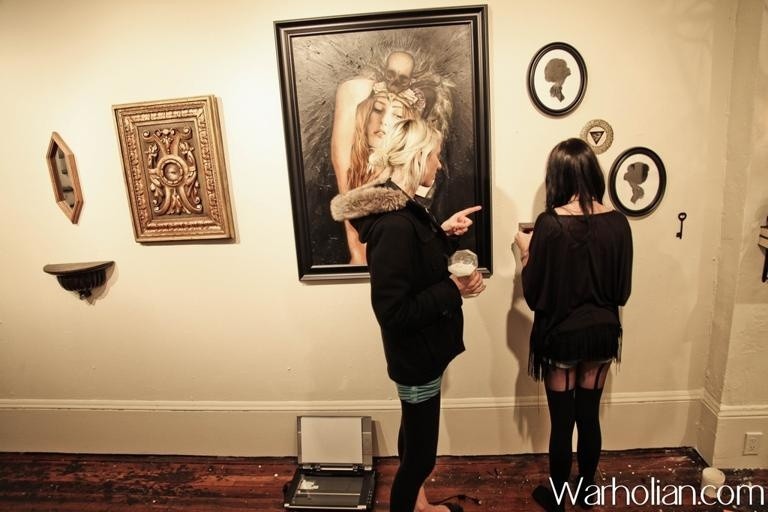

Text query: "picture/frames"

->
[269,4,493,288]
[528,40,669,218]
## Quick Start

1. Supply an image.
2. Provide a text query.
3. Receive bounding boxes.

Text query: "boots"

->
[412,482,451,512]
[576,475,596,508]
[531,485,566,512]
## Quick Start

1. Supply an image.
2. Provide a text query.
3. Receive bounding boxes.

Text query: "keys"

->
[676,212,687,239]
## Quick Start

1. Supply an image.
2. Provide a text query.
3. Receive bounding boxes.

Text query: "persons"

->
[329,77,435,266]
[329,115,488,512]
[511,137,634,511]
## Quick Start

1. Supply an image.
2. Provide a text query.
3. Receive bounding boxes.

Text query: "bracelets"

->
[520,249,529,262]
